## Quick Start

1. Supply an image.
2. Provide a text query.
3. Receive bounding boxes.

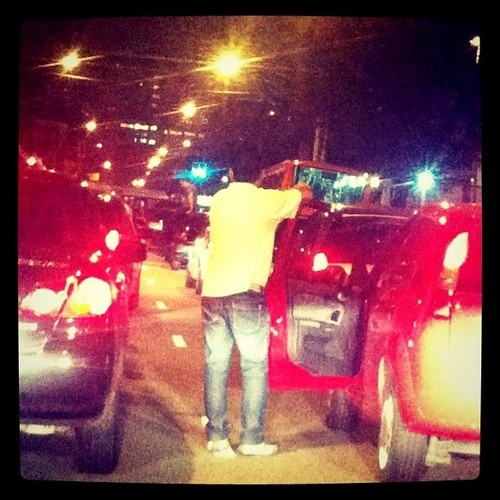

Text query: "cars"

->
[167,213,210,270]
[277,206,411,265]
[17,169,147,470]
[262,201,481,486]
[188,224,212,295]
[96,184,184,304]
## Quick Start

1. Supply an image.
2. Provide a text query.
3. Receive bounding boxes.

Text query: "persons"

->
[201,145,314,459]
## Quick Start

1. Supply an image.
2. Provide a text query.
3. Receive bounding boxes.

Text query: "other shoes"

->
[237,443,278,457]
[208,439,236,458]
